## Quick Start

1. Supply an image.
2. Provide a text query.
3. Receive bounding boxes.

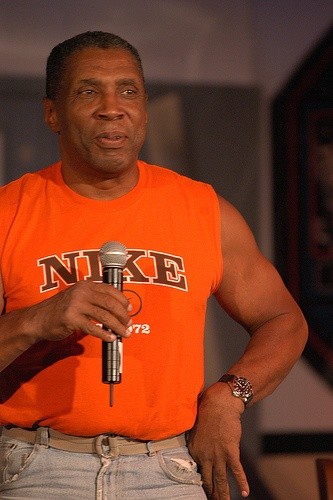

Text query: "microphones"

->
[99,241,128,385]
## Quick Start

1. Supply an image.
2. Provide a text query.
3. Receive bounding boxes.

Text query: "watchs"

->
[218,374,254,409]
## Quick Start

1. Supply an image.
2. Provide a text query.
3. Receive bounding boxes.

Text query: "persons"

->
[0,32,309,500]
